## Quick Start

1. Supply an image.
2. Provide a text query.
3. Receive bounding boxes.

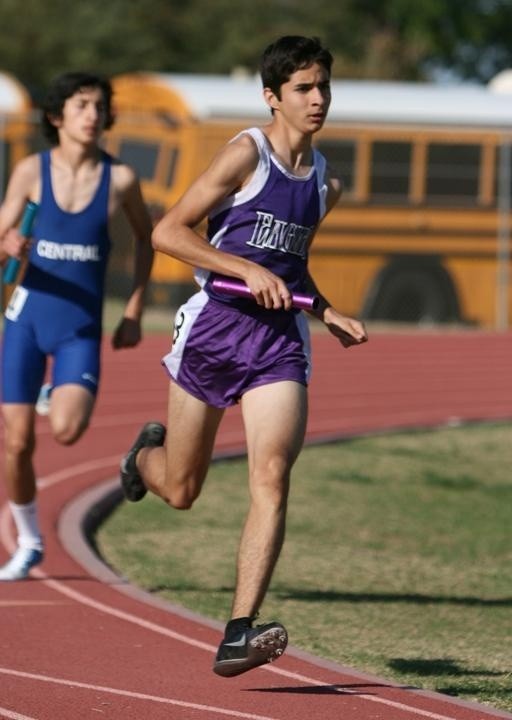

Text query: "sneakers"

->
[119,421,167,503]
[0,537,45,581]
[212,622,289,679]
[34,382,56,417]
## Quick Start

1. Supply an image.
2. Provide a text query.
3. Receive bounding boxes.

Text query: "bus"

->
[0,71,512,329]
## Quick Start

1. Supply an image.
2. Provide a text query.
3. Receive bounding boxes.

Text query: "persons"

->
[119,35,371,676]
[1,68,154,580]
